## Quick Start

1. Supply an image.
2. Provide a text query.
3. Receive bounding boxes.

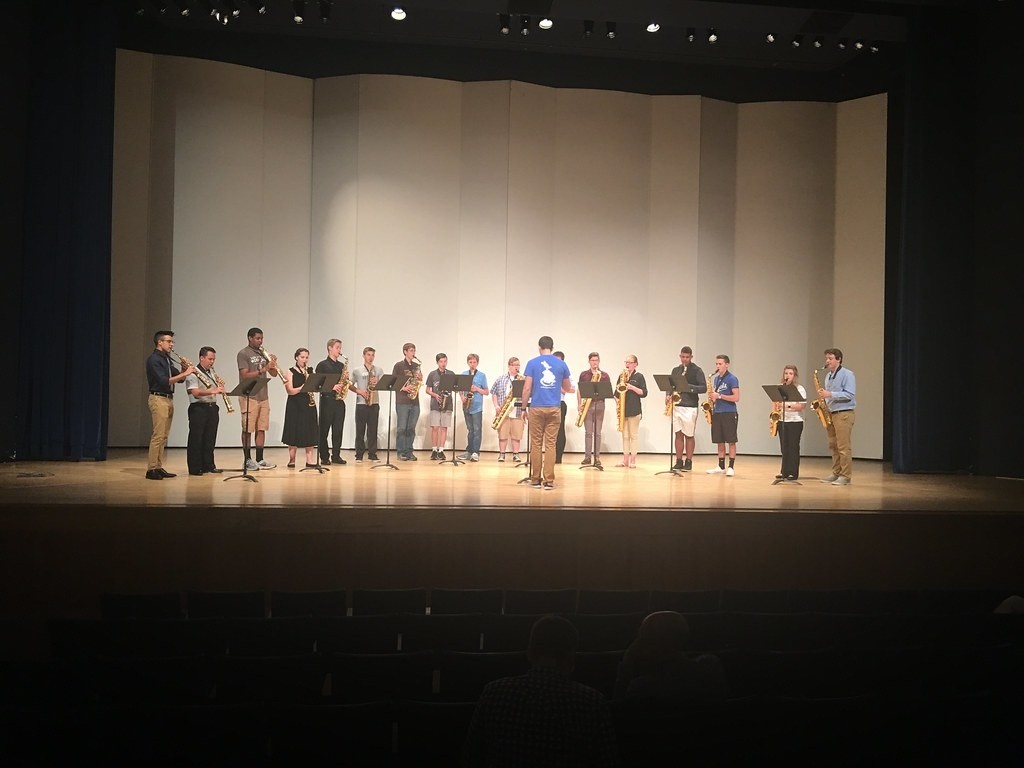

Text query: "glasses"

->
[162,340,173,343]
[624,361,634,364]
[511,364,520,366]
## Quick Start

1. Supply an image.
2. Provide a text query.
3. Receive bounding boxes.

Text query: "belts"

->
[207,403,216,406]
[151,392,172,398]
[832,410,850,413]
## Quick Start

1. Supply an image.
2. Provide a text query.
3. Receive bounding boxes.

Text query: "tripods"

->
[369,374,410,470]
[578,381,614,472]
[512,380,530,467]
[223,377,271,482]
[762,385,807,485]
[653,375,693,477]
[438,373,474,466]
[299,373,340,475]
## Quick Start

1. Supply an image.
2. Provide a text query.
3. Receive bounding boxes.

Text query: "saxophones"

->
[701,369,719,426]
[211,364,235,414]
[303,363,317,407]
[438,367,451,410]
[664,362,688,416]
[260,344,290,385]
[491,370,525,430]
[364,361,378,406]
[406,356,425,400]
[463,367,475,411]
[616,366,634,432]
[170,348,213,390]
[810,364,834,429]
[575,364,602,428]
[769,378,789,437]
[334,353,355,401]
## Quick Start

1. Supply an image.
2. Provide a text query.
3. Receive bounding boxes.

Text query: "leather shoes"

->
[332,457,346,464]
[158,468,175,477]
[321,460,330,464]
[204,468,223,473]
[147,471,163,480]
[191,470,203,475]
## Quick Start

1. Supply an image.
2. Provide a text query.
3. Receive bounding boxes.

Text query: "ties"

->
[167,358,174,392]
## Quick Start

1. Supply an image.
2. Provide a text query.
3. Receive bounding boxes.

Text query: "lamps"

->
[583,19,595,38]
[704,24,721,49]
[605,21,617,40]
[854,39,866,49]
[386,4,408,24]
[686,27,696,49]
[251,0,267,17]
[760,32,780,49]
[645,16,664,34]
[837,37,849,51]
[813,36,825,49]
[203,0,241,27]
[537,16,554,32]
[870,40,885,52]
[154,0,168,15]
[289,0,306,25]
[132,0,146,17]
[177,0,192,17]
[519,15,531,36]
[317,0,332,24]
[790,33,805,49]
[498,13,512,36]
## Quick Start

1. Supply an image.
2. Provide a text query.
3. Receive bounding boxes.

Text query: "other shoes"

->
[456,449,851,489]
[407,452,417,461]
[288,464,295,467]
[356,456,363,463]
[306,463,321,467]
[368,453,381,463]
[397,452,408,461]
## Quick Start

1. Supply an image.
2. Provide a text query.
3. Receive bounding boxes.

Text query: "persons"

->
[613,610,739,768]
[315,339,350,465]
[237,328,278,471]
[454,354,489,461]
[349,347,386,462]
[464,615,621,768]
[392,343,423,461]
[490,357,526,461]
[185,347,225,476]
[665,347,707,472]
[281,348,322,468]
[613,354,647,469]
[818,349,856,485]
[425,353,455,460]
[706,355,739,476]
[772,365,806,480]
[146,331,196,480]
[521,336,571,490]
[551,351,575,464]
[577,352,608,465]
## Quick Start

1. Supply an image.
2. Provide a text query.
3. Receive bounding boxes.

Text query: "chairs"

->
[0,589,1024,768]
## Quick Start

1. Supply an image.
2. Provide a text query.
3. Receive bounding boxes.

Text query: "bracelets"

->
[719,394,721,400]
[521,408,526,411]
[259,369,262,375]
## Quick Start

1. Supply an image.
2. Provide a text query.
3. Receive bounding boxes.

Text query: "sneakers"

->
[431,450,437,459]
[243,458,258,471]
[257,459,275,469]
[438,451,446,461]
